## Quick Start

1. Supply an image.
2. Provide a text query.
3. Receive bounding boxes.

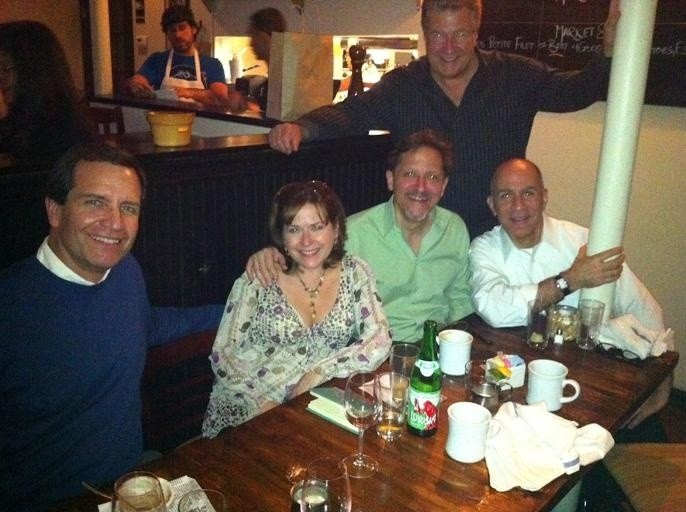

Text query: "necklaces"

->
[293,263,328,327]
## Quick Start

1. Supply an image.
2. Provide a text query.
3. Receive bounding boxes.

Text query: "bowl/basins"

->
[142,110,196,149]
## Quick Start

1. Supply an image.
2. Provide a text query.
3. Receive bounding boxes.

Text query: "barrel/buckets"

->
[147,109,196,147]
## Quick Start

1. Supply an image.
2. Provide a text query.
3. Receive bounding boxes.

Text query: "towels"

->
[484,401,615,494]
[590,313,676,361]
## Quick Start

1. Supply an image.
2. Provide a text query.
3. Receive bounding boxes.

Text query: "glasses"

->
[594,341,645,370]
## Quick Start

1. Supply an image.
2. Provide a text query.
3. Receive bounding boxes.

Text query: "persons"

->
[0,134,228,512]
[200,177,392,440]
[268,0,621,239]
[246,127,474,356]
[468,159,674,431]
[0,20,96,171]
[228,8,285,112]
[119,5,230,110]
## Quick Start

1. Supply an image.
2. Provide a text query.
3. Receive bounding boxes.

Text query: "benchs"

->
[140,327,223,460]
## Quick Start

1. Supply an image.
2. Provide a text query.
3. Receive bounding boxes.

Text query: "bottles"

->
[549,304,580,342]
[346,44,367,103]
[405,319,443,439]
[229,51,243,85]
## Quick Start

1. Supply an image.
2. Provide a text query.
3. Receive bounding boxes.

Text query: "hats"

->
[161,5,191,24]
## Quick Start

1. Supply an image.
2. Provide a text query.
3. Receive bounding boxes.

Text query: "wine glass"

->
[336,368,384,479]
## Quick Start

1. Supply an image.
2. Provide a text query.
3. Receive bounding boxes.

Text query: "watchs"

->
[553,276,570,297]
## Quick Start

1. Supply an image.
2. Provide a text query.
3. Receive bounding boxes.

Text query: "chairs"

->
[84,106,125,137]
[576,442,686,512]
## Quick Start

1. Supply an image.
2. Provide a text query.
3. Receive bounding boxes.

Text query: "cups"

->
[434,328,477,376]
[486,354,526,392]
[388,343,420,404]
[526,297,556,352]
[372,373,411,443]
[443,401,502,463]
[106,455,355,512]
[463,359,514,412]
[527,357,582,415]
[577,297,606,352]
